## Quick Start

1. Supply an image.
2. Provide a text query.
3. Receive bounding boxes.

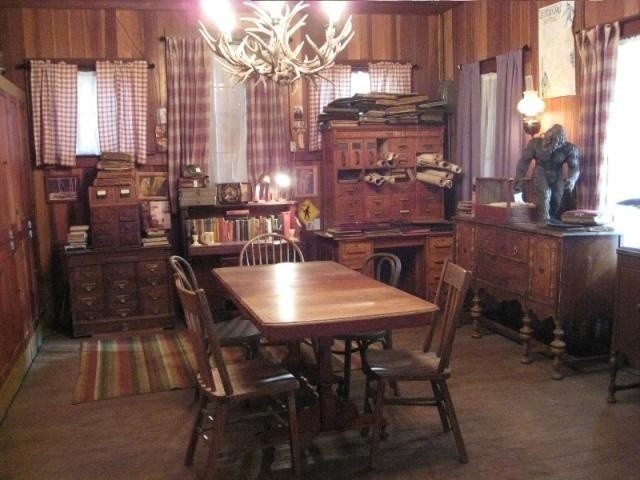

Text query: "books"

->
[544,209,617,232]
[89,151,135,187]
[143,241,169,246]
[146,229,165,235]
[141,235,169,242]
[316,91,445,124]
[326,219,432,239]
[184,210,296,246]
[176,175,218,206]
[63,222,90,252]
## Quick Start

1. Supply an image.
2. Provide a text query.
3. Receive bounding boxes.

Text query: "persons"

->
[512,122,582,219]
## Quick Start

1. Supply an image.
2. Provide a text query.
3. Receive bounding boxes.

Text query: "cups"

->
[203,231,216,244]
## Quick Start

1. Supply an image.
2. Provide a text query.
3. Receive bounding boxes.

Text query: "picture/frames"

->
[44,175,81,204]
[293,166,319,197]
[137,172,170,201]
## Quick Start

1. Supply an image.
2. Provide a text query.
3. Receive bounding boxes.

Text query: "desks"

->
[223,259,440,480]
[316,226,455,313]
[606,235,640,405]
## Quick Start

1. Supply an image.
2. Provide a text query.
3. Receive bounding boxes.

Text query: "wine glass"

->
[192,235,200,244]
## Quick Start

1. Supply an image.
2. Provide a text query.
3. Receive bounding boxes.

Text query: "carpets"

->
[72,321,383,405]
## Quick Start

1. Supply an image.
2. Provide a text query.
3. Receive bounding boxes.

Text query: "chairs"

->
[362,258,473,470]
[170,269,306,480]
[319,254,402,396]
[240,232,308,267]
[168,254,262,369]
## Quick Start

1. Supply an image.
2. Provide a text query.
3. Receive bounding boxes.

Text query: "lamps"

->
[515,75,545,137]
[185,0,359,92]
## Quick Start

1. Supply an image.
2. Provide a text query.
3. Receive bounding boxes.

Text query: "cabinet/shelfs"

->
[179,200,315,324]
[322,115,450,229]
[87,184,142,251]
[450,214,621,379]
[61,244,173,334]
